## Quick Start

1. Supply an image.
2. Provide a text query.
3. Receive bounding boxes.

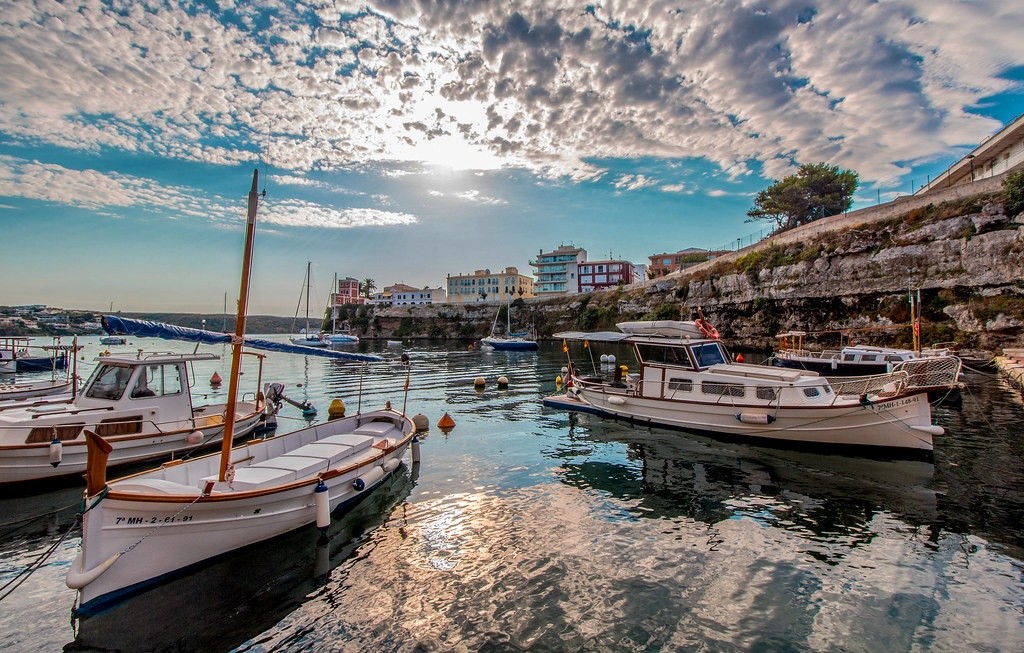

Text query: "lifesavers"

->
[694,318,720,339]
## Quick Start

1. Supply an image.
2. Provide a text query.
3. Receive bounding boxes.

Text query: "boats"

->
[759,289,998,392]
[541,321,946,461]
[0,341,285,494]
[99,334,127,345]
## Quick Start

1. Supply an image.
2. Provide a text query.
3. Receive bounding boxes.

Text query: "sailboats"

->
[315,271,360,342]
[66,167,414,621]
[288,260,333,348]
[479,279,540,351]
